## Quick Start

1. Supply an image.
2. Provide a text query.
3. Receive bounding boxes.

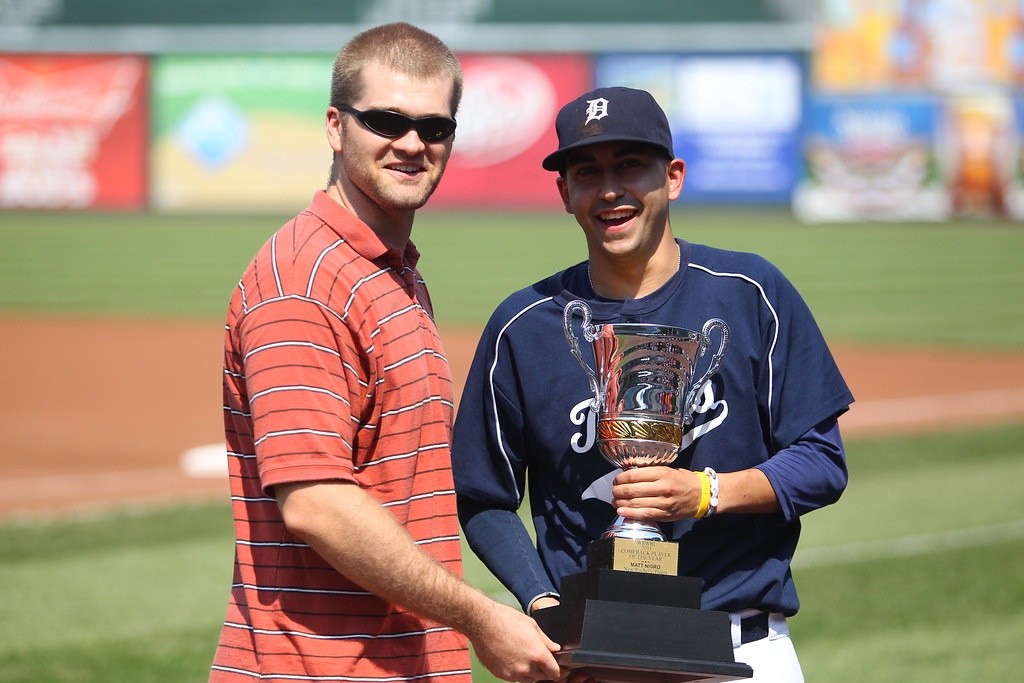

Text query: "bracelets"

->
[694,466,722,519]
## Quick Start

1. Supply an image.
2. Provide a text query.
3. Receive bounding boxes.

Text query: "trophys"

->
[529,299,757,679]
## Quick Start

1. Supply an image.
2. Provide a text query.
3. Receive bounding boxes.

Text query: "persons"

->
[452,86,855,683]
[216,21,563,683]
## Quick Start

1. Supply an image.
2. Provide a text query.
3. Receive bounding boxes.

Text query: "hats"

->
[542,86,677,172]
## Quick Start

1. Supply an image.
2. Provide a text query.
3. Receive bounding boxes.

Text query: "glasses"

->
[336,103,458,143]
